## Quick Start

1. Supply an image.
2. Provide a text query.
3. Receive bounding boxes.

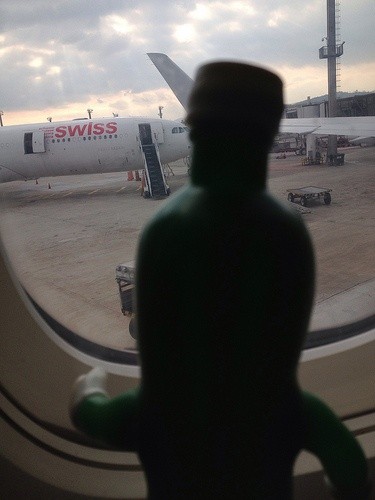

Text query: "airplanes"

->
[0,52,375,186]
